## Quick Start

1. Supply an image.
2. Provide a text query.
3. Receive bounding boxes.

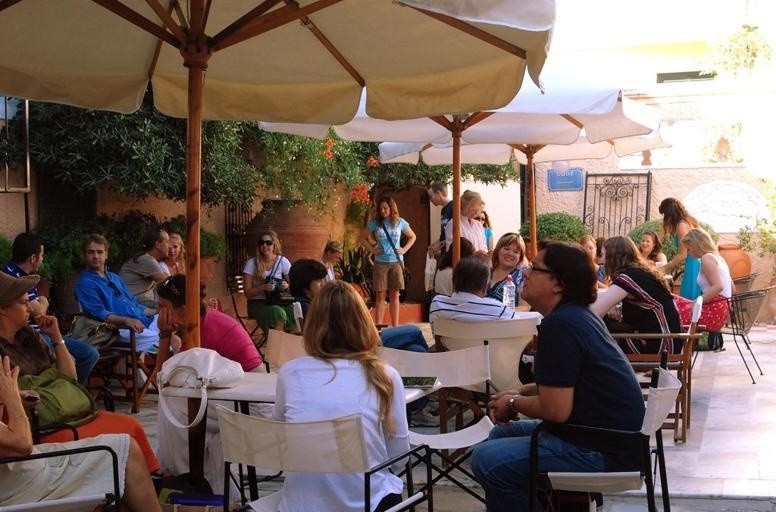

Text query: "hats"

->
[0,268,41,307]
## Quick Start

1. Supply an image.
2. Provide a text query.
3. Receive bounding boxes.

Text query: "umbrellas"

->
[379,124,679,260]
[0,2,557,480]
[253,81,655,266]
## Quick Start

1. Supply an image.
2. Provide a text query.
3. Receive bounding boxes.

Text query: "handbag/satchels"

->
[697,330,724,351]
[157,348,245,429]
[64,316,117,352]
[20,363,100,435]
[264,273,296,306]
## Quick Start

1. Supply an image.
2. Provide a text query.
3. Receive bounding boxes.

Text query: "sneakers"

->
[408,408,441,428]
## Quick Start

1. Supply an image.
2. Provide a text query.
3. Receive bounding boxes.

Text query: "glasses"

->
[164,275,182,298]
[257,240,273,245]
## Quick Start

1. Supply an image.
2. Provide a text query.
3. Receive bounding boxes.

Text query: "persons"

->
[469,240,647,511]
[1,225,270,511]
[245,280,412,511]
[241,180,739,426]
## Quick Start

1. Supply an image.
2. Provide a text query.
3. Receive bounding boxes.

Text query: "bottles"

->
[503,275,515,312]
